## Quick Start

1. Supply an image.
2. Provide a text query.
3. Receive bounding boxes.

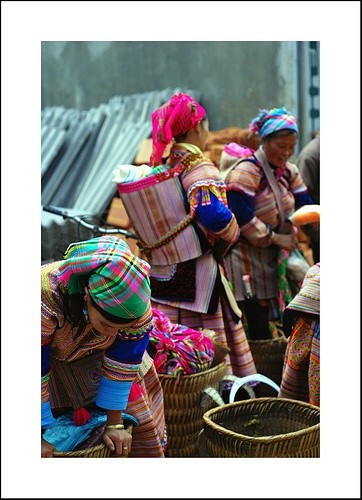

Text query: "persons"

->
[41,92,321,458]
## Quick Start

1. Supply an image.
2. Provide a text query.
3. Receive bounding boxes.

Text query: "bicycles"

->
[42,205,143,242]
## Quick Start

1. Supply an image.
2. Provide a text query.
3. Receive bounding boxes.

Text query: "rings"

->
[123,446,128,449]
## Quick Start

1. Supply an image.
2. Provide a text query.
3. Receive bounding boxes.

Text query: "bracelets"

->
[104,424,125,429]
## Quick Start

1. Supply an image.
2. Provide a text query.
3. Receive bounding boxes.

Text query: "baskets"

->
[202,398,320,458]
[157,358,228,457]
[53,424,132,458]
[248,330,288,374]
